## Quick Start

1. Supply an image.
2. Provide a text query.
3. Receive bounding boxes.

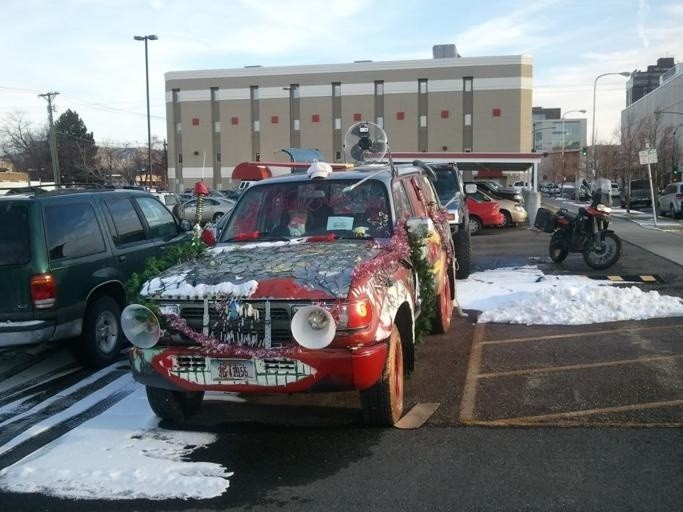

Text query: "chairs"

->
[271,208,327,235]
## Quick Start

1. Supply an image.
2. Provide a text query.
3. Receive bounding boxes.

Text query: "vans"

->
[0,182,198,368]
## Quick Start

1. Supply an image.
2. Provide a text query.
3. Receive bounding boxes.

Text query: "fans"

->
[343,119,387,167]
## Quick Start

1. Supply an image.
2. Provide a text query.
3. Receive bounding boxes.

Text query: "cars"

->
[463,176,531,235]
[152,187,239,233]
[540,177,683,219]
[362,159,472,281]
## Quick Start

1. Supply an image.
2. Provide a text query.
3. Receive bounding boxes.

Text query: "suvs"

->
[116,159,459,439]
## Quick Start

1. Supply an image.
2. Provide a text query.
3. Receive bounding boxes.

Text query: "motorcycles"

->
[534,178,624,270]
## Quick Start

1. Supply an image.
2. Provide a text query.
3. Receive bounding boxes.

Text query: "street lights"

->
[134,33,159,189]
[590,71,630,167]
[560,109,586,196]
